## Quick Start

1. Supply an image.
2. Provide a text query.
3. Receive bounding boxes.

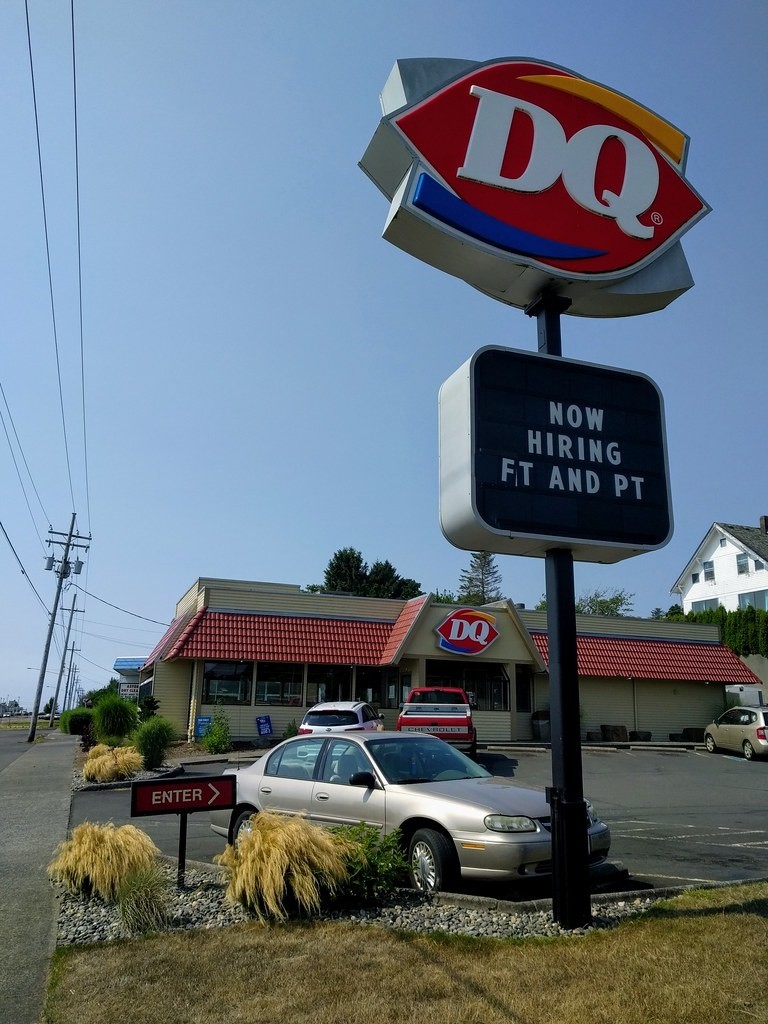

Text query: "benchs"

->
[279,764,338,783]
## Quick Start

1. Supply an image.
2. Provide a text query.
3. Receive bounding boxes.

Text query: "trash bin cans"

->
[532,710,551,742]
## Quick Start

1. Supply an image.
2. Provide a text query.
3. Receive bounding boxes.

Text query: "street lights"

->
[28,555,83,743]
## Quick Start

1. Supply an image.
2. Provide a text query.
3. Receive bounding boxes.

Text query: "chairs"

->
[382,752,403,782]
[328,755,358,783]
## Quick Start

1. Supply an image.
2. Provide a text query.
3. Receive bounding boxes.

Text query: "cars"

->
[208,730,611,894]
[3,713,10,717]
[39,713,60,720]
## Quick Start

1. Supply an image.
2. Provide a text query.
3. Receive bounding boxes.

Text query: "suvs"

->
[704,705,768,761]
[297,701,384,761]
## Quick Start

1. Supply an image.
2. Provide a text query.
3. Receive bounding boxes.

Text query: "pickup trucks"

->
[397,687,477,756]
